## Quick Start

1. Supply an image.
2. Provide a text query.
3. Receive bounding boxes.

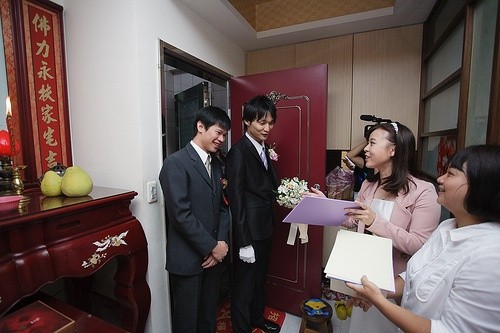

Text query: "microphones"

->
[360,115,378,122]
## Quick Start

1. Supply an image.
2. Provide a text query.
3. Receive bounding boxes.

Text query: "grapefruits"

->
[41,171,63,196]
[61,166,92,196]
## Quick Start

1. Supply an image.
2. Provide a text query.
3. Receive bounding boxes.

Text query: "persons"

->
[349,144,500,333]
[301,122,442,333]
[223,96,281,333]
[160,106,231,333]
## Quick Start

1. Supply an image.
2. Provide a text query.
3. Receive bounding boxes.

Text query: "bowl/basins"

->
[0,195,21,213]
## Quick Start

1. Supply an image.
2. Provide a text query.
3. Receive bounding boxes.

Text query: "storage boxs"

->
[0,300,76,332]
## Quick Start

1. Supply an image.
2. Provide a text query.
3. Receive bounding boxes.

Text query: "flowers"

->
[272,177,309,209]
[268,147,280,161]
[220,178,227,190]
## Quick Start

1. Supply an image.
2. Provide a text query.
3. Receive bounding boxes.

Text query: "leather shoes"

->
[257,316,280,333]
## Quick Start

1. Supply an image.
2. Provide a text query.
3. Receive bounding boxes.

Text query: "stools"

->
[299,298,333,333]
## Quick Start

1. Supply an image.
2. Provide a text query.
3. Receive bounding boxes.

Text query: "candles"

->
[6,96,18,166]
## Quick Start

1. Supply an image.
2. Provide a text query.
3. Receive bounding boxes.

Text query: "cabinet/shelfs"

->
[246,22,424,150]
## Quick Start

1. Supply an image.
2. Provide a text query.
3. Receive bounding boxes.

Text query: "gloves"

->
[238,244,256,263]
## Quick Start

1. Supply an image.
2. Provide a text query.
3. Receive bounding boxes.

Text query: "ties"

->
[205,157,212,180]
[261,149,269,171]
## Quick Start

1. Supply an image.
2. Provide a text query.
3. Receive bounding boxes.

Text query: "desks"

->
[0,184,151,333]
